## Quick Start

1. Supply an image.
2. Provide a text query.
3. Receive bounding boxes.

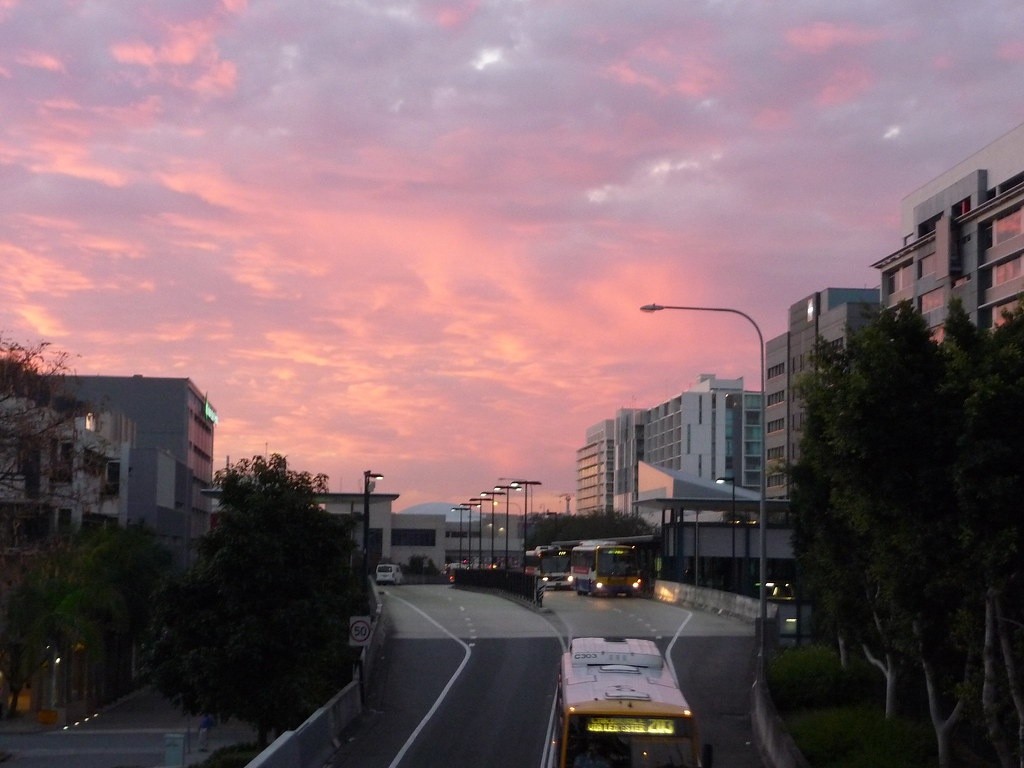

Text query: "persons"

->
[573,740,612,768]
[198,713,214,752]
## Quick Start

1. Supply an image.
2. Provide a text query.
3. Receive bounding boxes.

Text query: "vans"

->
[375,564,403,585]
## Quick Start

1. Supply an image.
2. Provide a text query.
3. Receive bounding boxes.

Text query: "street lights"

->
[494,484,522,574]
[510,480,544,575]
[451,506,472,567]
[361,469,385,593]
[640,304,768,645]
[469,497,494,567]
[478,493,507,571]
[459,503,482,568]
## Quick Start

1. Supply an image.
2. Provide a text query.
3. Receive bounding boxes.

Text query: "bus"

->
[524,546,576,591]
[569,541,642,598]
[553,634,713,768]
